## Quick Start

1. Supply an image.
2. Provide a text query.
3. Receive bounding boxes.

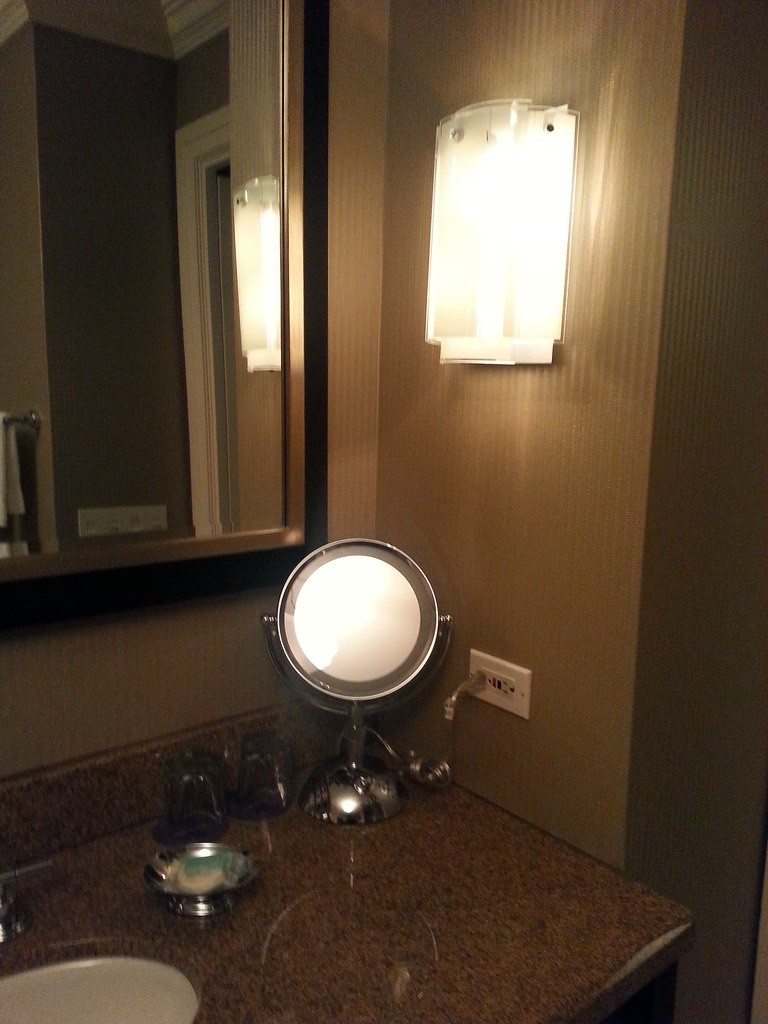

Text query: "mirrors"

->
[0,0,332,637]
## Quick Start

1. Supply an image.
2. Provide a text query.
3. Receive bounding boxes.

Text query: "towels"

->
[0,410,30,557]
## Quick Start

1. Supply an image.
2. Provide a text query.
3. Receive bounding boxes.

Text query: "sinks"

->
[1,937,206,1022]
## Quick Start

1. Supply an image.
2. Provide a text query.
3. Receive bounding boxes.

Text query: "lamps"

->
[423,101,579,364]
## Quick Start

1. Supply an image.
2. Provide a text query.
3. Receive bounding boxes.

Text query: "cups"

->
[233,736,288,813]
[163,762,227,843]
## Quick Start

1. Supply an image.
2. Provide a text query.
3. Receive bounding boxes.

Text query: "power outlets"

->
[467,647,531,721]
